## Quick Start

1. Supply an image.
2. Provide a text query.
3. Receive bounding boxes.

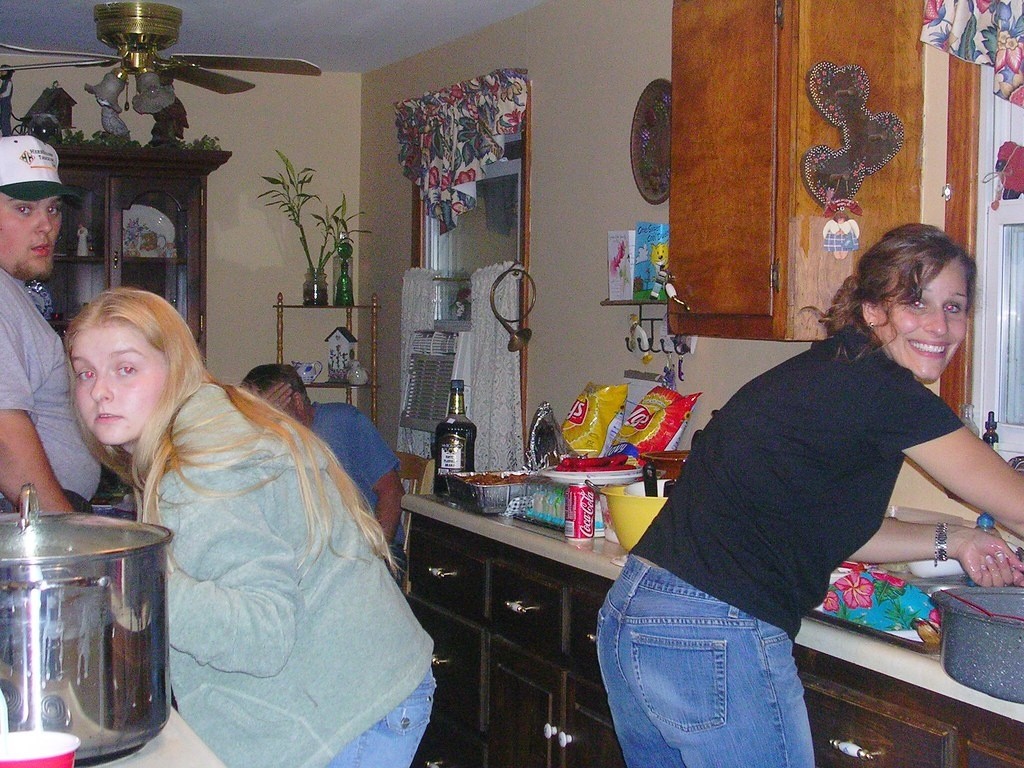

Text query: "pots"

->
[0,481,173,760]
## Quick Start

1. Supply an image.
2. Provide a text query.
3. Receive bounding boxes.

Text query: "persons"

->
[0,134,102,515]
[596,223,1024,767]
[240,364,407,591]
[64,286,437,768]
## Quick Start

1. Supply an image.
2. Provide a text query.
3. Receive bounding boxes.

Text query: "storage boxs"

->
[632,222,669,301]
[607,231,636,300]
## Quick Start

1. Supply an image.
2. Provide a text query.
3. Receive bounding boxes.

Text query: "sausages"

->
[555,454,637,472]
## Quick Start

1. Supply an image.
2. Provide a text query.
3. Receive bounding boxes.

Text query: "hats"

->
[0,135,77,201]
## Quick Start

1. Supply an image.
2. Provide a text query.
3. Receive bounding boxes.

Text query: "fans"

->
[0,0,323,92]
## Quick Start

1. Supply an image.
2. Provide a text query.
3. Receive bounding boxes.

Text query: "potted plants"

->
[262,150,373,305]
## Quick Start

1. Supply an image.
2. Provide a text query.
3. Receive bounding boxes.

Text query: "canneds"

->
[563,483,596,542]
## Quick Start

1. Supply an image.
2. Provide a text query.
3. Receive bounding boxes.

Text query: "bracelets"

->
[934,523,948,567]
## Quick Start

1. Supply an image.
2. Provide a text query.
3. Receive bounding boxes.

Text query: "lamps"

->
[81,67,176,116]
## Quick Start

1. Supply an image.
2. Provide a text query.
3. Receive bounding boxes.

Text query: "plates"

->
[119,204,176,260]
[23,278,54,322]
[538,464,641,487]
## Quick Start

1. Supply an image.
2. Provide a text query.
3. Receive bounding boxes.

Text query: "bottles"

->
[983,412,999,454]
[957,403,979,442]
[436,379,478,496]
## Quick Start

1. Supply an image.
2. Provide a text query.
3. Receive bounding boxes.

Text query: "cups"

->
[0,731,81,768]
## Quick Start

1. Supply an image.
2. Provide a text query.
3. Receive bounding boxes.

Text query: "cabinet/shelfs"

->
[668,0,924,336]
[31,139,234,370]
[271,289,381,426]
[405,513,1024,768]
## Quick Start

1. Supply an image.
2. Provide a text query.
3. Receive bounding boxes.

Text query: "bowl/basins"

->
[642,448,692,481]
[600,485,674,555]
[932,585,1023,706]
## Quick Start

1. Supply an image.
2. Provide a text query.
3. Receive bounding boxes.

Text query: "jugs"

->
[290,360,322,384]
